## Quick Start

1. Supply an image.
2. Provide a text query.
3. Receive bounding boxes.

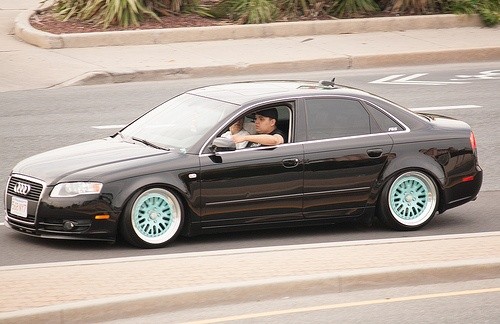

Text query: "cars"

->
[4,79,482,249]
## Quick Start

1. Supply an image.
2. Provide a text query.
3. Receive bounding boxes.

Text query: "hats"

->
[256,107,278,120]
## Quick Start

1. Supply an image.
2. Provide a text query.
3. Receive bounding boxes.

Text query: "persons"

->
[227,108,284,148]
[220,115,250,150]
